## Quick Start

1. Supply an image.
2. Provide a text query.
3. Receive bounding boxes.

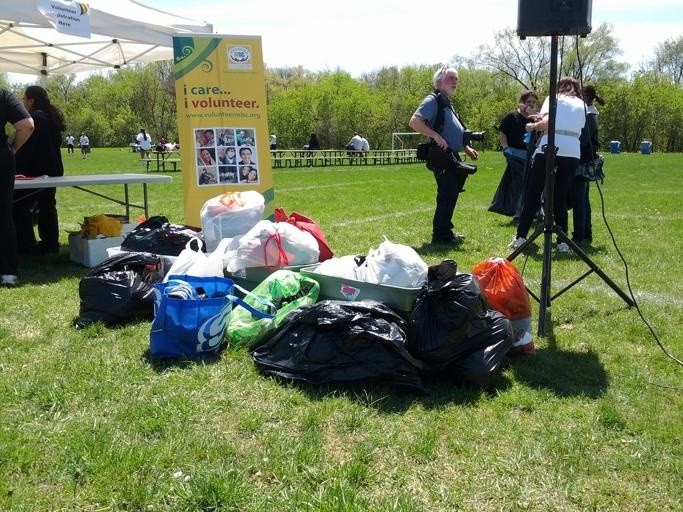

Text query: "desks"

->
[13,172,172,225]
[152,148,428,173]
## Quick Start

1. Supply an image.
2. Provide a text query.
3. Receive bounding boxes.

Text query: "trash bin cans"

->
[641,141,652,154]
[610,141,621,154]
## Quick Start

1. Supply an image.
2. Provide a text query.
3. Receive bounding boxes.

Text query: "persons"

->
[346,132,362,156]
[66,133,75,155]
[1,84,35,285]
[499,76,608,252]
[14,85,65,258]
[270,132,276,158]
[80,131,89,159]
[156,136,180,151]
[195,128,257,186]
[359,134,369,156]
[137,128,152,159]
[409,65,479,246]
[301,132,320,156]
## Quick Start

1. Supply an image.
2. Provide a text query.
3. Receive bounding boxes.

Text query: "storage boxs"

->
[68,233,123,268]
[244,259,426,321]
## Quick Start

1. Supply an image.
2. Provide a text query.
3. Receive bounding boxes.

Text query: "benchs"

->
[138,155,428,173]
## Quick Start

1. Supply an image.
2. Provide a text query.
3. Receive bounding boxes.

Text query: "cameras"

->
[463,129,487,145]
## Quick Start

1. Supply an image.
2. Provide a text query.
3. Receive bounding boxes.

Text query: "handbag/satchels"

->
[149,275,234,356]
[582,154,605,185]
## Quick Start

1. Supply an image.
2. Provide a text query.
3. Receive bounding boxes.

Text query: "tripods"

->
[506,32,636,338]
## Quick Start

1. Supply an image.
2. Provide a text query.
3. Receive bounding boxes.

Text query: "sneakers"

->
[433,232,464,243]
[558,242,568,253]
[508,233,529,248]
[38,241,59,252]
[1,274,18,285]
